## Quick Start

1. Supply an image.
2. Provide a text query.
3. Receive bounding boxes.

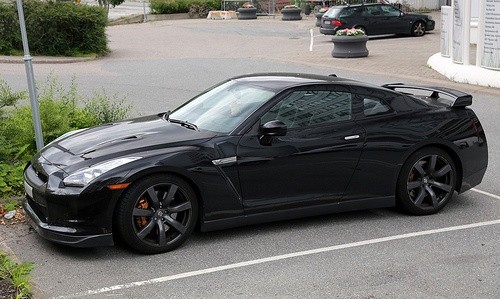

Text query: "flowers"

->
[319,7,329,13]
[284,5,298,8]
[335,28,365,36]
[242,3,254,8]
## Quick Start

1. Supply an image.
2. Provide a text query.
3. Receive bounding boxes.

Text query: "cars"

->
[320,2,436,36]
[20,71,489,254]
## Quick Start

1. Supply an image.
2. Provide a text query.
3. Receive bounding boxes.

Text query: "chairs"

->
[262,102,299,134]
